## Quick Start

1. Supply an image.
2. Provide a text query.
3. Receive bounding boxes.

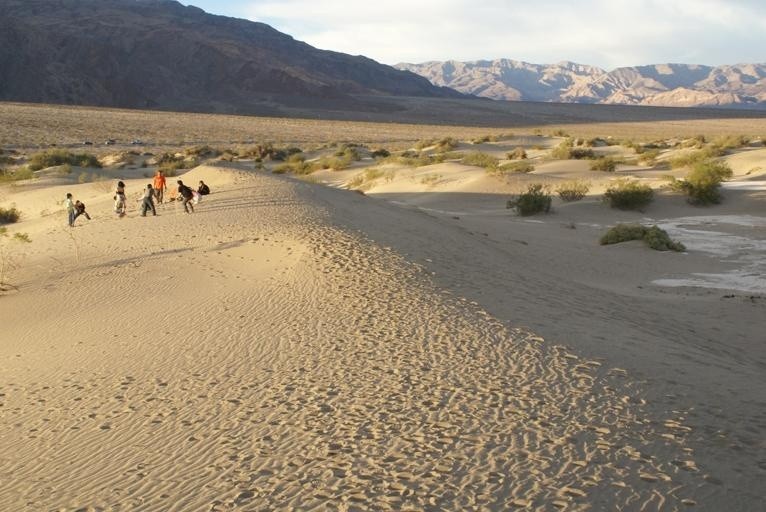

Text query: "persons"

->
[113,170,210,216]
[66,193,77,227]
[74,200,91,219]
